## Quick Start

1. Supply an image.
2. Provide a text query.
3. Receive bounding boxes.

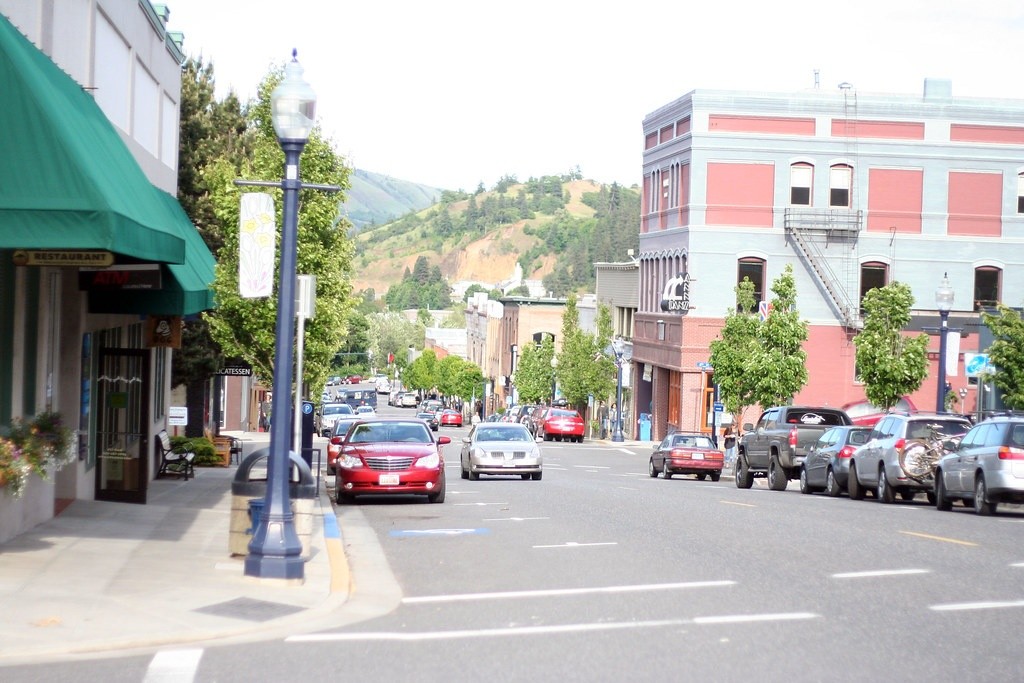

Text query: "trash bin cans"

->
[638,413,652,441]
[225,447,317,561]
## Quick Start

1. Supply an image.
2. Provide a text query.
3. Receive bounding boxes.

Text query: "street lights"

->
[241,49,317,580]
[612,334,625,442]
[935,272,957,414]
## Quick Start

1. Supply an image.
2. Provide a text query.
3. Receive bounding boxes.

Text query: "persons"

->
[472,411,481,423]
[597,401,618,439]
[723,424,736,468]
[950,395,959,414]
[261,395,272,432]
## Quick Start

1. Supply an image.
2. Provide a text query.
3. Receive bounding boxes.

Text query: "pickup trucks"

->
[732,405,875,492]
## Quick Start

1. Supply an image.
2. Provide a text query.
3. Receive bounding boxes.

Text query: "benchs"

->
[156,429,194,481]
[205,428,232,467]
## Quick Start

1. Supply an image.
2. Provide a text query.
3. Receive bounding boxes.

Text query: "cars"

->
[315,369,463,477]
[839,396,918,426]
[492,403,586,445]
[648,434,723,482]
[799,426,916,499]
[330,417,452,503]
[460,422,544,481]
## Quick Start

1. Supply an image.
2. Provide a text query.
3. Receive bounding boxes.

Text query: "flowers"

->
[0,411,77,501]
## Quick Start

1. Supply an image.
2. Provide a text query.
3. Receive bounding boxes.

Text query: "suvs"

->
[846,410,977,508]
[929,409,1024,516]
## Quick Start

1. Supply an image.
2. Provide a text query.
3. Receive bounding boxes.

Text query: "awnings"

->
[86,183,221,315]
[0,13,186,269]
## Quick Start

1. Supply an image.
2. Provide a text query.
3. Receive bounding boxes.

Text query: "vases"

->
[0,470,8,488]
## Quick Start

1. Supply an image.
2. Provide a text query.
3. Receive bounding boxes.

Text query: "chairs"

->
[230,437,243,464]
[478,432,490,441]
